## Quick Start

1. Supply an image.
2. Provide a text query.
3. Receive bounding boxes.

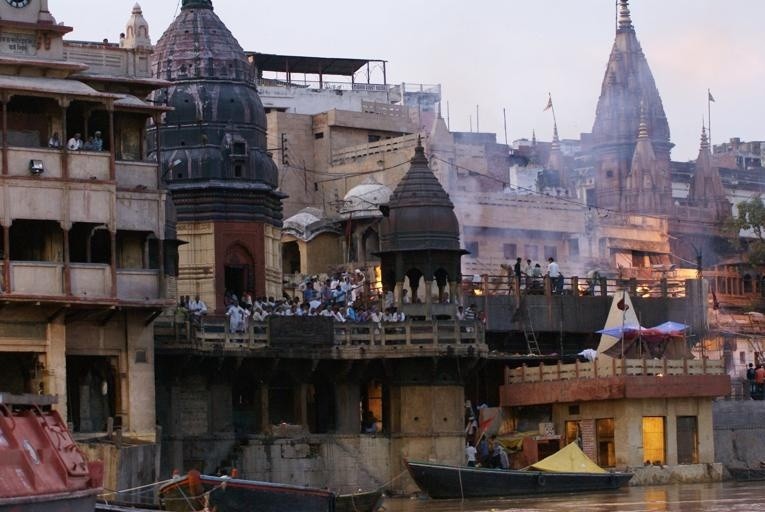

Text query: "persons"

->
[463,397,490,441]
[754,363,765,393]
[479,433,489,467]
[82,135,96,152]
[456,304,486,333]
[178,294,207,337]
[403,289,449,321]
[224,289,300,344]
[747,362,755,379]
[93,131,105,152]
[464,440,478,467]
[296,269,405,345]
[118,33,126,48]
[66,133,83,152]
[489,434,505,469]
[361,411,378,432]
[515,257,563,294]
[48,132,60,149]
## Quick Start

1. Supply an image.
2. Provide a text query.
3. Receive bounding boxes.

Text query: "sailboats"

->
[405,444,635,499]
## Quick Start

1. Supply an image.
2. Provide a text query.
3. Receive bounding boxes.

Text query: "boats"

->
[0,394,105,512]
[158,467,387,512]
[725,466,765,483]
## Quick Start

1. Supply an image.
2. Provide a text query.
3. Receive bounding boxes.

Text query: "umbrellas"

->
[543,96,553,111]
[708,92,716,103]
